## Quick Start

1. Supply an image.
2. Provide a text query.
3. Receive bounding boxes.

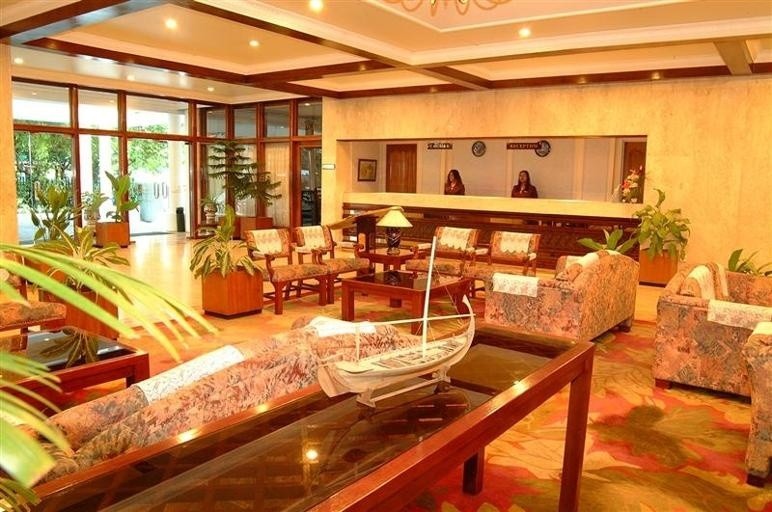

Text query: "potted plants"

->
[187,188,267,317]
[94,169,143,250]
[17,181,92,284]
[628,188,691,288]
[204,139,281,241]
[23,220,133,338]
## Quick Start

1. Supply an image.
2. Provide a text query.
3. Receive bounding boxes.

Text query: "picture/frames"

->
[357,157,378,181]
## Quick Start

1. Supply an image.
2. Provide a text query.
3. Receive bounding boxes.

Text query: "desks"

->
[0,323,597,512]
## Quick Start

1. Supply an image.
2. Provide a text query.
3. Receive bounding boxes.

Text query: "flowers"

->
[613,168,643,203]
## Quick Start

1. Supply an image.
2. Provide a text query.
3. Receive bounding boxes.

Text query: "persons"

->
[510,170,537,198]
[444,169,465,195]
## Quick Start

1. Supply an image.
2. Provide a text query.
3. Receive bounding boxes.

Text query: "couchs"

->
[737,320,772,489]
[483,248,642,342]
[651,259,772,400]
[2,315,423,485]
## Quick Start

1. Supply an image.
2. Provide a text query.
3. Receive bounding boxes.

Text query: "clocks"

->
[534,140,550,157]
[472,142,486,157]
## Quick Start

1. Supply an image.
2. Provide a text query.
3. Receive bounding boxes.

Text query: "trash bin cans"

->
[176,206,184,233]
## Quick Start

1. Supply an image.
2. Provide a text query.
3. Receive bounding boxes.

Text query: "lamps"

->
[374,210,414,255]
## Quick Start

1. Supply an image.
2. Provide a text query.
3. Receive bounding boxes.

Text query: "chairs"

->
[244,226,543,314]
